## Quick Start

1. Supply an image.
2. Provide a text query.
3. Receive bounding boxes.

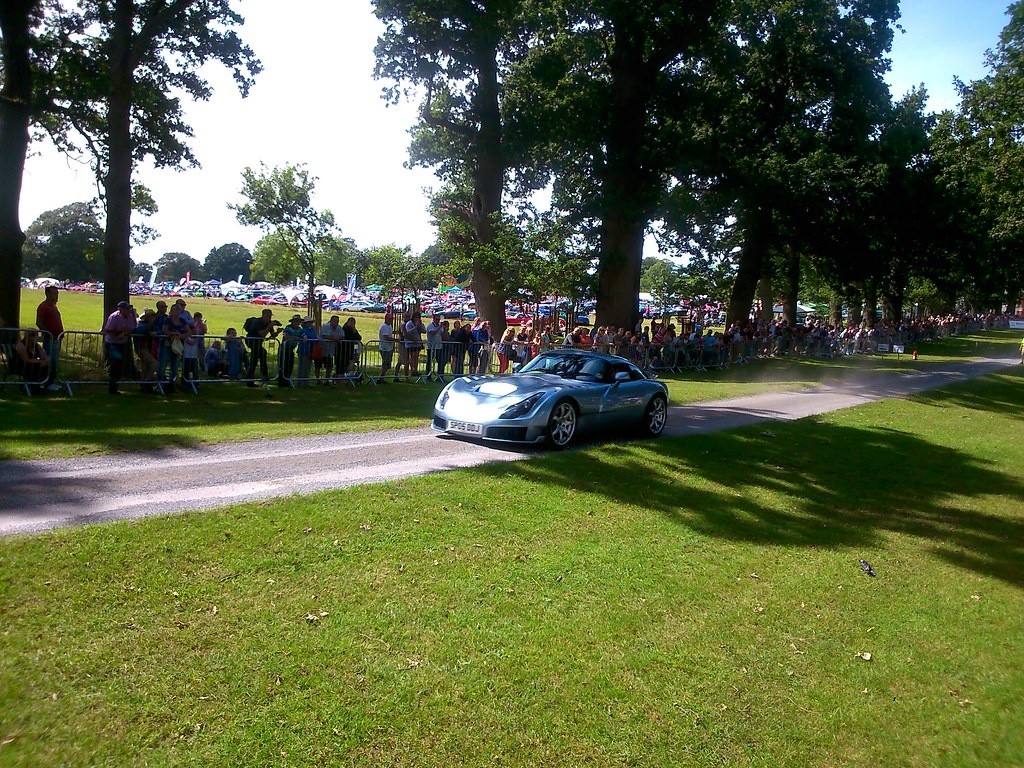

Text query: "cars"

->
[50,279,687,325]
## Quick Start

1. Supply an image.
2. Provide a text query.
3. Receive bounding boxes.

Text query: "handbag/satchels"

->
[310,343,323,359]
[171,336,184,356]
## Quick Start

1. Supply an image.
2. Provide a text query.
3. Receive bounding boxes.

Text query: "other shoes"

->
[110,391,121,395]
[246,383,259,387]
[48,383,62,391]
[405,380,411,383]
[377,379,387,384]
[325,381,329,386]
[195,384,201,389]
[411,371,422,376]
[393,378,403,382]
[316,381,322,385]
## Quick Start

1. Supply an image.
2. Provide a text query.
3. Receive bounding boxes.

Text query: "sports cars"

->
[430,348,670,448]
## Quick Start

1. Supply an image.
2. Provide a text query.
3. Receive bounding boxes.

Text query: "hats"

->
[118,301,133,309]
[386,314,393,319]
[304,316,316,323]
[140,310,158,320]
[289,314,304,322]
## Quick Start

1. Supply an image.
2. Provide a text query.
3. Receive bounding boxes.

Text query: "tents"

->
[19,277,881,321]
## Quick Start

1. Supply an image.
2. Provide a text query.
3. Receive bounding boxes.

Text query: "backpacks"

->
[244,317,261,332]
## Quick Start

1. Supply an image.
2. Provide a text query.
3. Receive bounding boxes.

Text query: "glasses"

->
[434,318,440,321]
[159,305,168,308]
[180,304,187,306]
[414,316,421,319]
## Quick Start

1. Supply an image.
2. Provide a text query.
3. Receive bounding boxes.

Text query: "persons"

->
[13,287,1024,396]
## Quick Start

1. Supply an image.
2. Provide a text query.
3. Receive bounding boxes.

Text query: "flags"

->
[136,267,356,301]
